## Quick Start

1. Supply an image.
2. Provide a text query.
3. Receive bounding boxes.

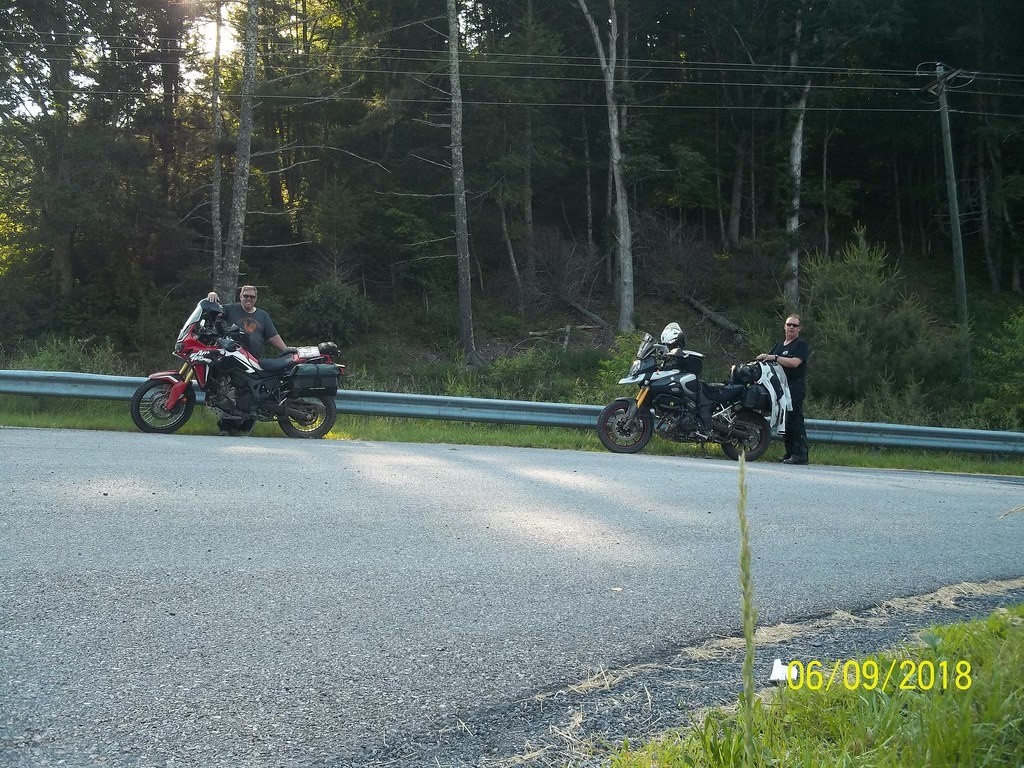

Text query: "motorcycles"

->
[130,295,345,440]
[595,333,784,462]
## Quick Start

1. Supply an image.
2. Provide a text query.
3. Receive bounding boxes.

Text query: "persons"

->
[757,313,809,465]
[206,285,287,436]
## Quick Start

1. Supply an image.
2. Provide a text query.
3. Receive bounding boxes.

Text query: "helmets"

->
[661,322,686,350]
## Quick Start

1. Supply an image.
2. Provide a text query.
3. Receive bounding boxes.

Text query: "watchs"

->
[775,355,778,362]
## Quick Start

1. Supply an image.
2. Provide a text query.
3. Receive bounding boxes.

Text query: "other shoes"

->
[777,456,790,462]
[783,456,808,465]
[220,430,230,436]
[247,419,256,436]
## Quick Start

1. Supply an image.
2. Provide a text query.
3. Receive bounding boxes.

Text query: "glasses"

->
[785,323,800,327]
[241,294,257,300]
